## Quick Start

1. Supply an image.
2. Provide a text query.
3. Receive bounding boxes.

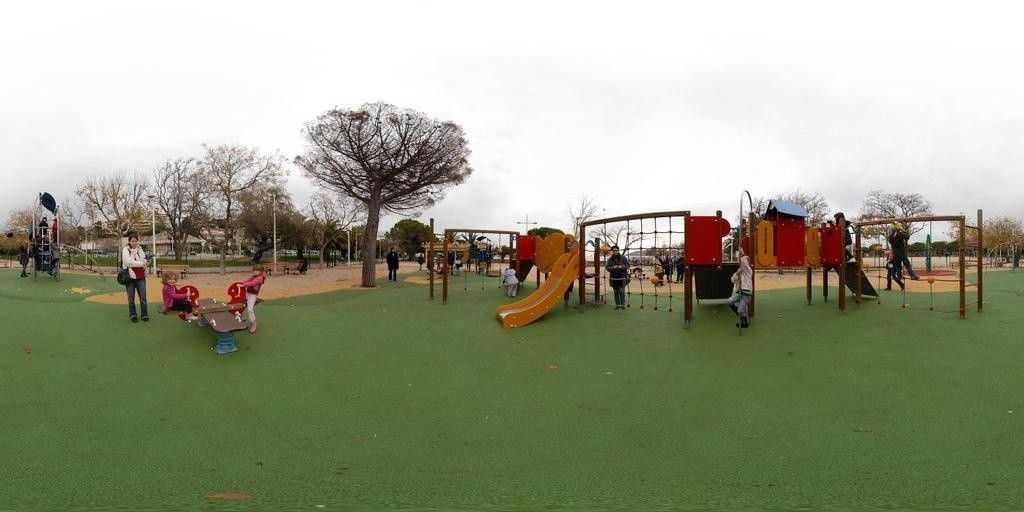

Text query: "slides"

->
[494,251,581,330]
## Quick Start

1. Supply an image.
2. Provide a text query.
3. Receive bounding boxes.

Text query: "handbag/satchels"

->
[117,268,131,284]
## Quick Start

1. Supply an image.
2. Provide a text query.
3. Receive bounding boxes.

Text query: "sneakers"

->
[131,315,149,323]
[249,322,257,333]
[736,317,748,328]
[185,313,198,320]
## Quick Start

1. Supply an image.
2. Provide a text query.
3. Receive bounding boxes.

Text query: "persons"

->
[29,217,60,268]
[122,234,150,323]
[456,255,462,270]
[503,267,519,297]
[827,212,859,261]
[448,253,454,275]
[883,250,904,291]
[606,245,631,309]
[728,247,753,328]
[417,253,425,270]
[653,251,685,287]
[237,263,266,333]
[386,247,400,281]
[889,223,920,281]
[20,245,29,277]
[161,270,199,320]
